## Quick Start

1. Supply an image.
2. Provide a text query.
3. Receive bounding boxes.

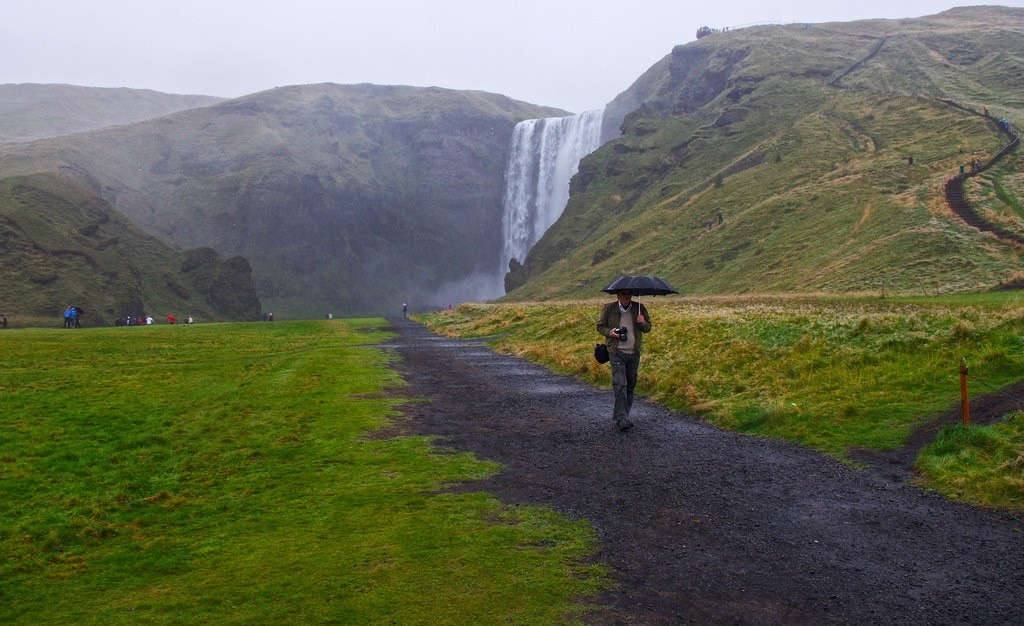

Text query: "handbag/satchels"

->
[595,344,609,364]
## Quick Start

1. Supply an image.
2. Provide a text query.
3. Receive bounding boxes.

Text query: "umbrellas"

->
[599,274,679,315]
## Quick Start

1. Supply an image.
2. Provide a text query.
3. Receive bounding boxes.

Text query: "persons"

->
[708,220,714,228]
[268,311,273,321]
[168,313,175,324]
[718,211,723,223]
[113,314,156,326]
[403,304,408,318]
[448,305,453,311]
[959,163,964,174]
[595,288,652,430]
[0,317,8,329]
[62,304,84,329]
[263,312,268,321]
[908,155,914,166]
[325,313,334,320]
[184,316,193,323]
[971,158,976,173]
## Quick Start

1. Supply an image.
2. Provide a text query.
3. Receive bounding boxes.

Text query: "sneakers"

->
[616,418,634,430]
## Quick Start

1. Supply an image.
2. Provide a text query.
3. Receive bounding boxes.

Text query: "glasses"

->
[618,292,632,296]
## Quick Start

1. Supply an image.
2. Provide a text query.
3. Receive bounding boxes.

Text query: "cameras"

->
[614,326,628,342]
